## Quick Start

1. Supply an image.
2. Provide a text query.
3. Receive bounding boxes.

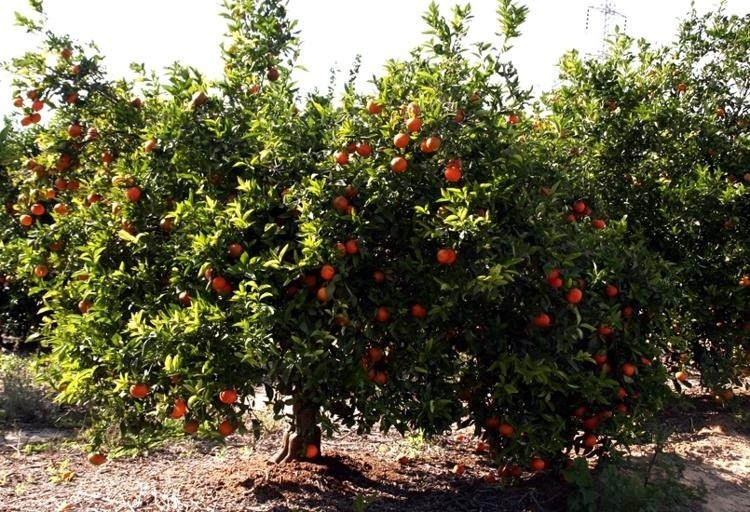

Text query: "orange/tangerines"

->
[13,65,750,483]
[61,49,72,60]
